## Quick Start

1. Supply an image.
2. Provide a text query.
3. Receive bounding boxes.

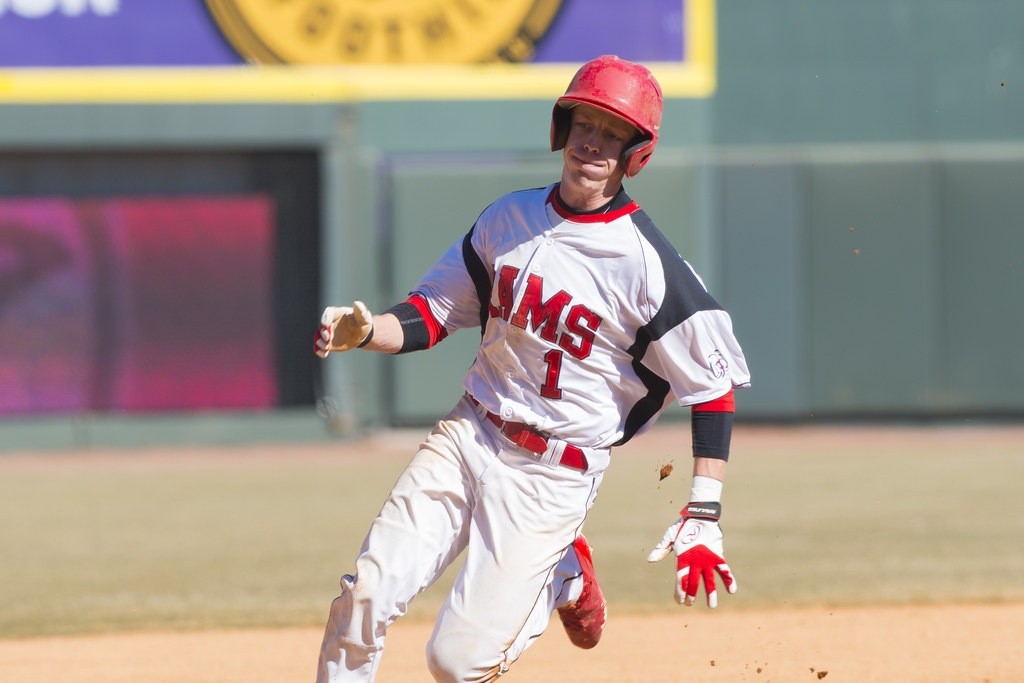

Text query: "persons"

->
[311,55,752,683]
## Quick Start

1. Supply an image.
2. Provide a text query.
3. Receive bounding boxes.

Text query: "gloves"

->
[313,301,373,359]
[648,501,737,609]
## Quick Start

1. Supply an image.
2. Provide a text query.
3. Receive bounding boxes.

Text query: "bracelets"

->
[356,326,373,347]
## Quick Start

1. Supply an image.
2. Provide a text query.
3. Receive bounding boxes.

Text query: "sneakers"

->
[556,533,607,650]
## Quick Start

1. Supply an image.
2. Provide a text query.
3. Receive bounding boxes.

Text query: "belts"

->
[470,395,588,470]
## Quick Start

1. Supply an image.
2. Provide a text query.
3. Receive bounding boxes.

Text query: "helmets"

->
[550,55,663,179]
